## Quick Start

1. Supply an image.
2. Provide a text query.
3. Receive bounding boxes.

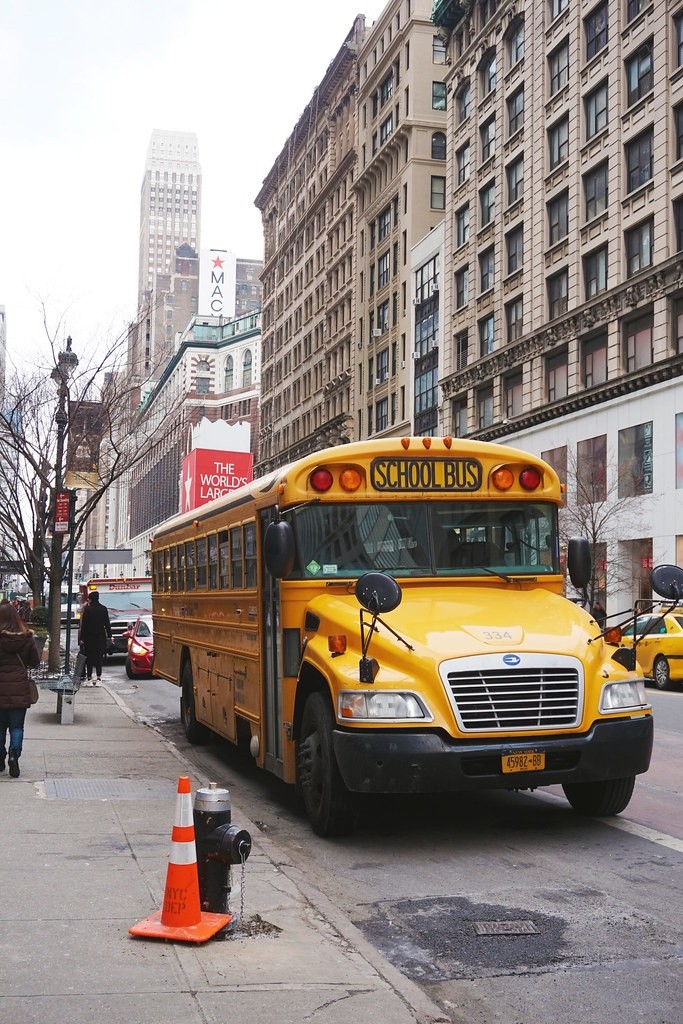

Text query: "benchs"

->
[40,654,88,725]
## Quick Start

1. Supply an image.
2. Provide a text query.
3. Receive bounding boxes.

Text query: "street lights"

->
[49,336,78,674]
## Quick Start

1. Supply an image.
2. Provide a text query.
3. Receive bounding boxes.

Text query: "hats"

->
[88,592,99,601]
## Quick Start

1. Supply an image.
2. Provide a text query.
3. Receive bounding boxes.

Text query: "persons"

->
[80,591,115,688]
[592,601,608,630]
[18,600,27,611]
[0,601,41,778]
[7,597,19,607]
[0,598,12,610]
[17,600,33,622]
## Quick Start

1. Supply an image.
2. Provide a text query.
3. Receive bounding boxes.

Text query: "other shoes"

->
[0,753,19,779]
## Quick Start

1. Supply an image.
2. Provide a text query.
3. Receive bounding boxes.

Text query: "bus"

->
[149,437,655,840]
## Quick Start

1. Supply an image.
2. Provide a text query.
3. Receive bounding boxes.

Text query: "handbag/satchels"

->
[28,679,39,706]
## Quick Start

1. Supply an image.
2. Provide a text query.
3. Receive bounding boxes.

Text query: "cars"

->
[123,614,155,679]
[75,578,153,664]
[606,612,683,690]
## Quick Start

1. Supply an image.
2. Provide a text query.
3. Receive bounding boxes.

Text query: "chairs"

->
[411,524,507,569]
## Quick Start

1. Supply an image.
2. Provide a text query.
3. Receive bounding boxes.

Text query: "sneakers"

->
[82,677,101,687]
